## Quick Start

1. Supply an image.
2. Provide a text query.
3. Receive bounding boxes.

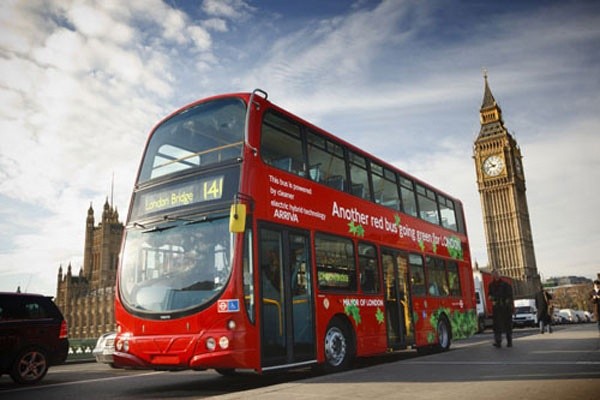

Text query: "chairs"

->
[442,217,448,227]
[424,213,436,223]
[326,175,344,189]
[270,154,292,171]
[428,281,439,296]
[301,162,322,184]
[351,183,364,197]
[404,206,413,214]
[382,199,401,212]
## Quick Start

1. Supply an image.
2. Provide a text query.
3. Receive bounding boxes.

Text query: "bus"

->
[112,89,480,376]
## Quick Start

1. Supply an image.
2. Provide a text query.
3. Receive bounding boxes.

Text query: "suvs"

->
[0,292,69,385]
[513,306,537,328]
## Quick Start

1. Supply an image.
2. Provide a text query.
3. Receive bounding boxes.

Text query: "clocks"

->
[482,155,504,178]
[515,157,523,174]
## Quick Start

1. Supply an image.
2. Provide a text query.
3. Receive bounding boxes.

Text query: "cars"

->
[92,332,122,369]
[551,307,595,323]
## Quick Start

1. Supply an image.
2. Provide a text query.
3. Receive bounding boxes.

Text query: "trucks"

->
[473,270,514,334]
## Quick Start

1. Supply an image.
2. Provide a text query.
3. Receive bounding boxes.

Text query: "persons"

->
[487,269,516,346]
[590,281,599,334]
[537,285,553,334]
